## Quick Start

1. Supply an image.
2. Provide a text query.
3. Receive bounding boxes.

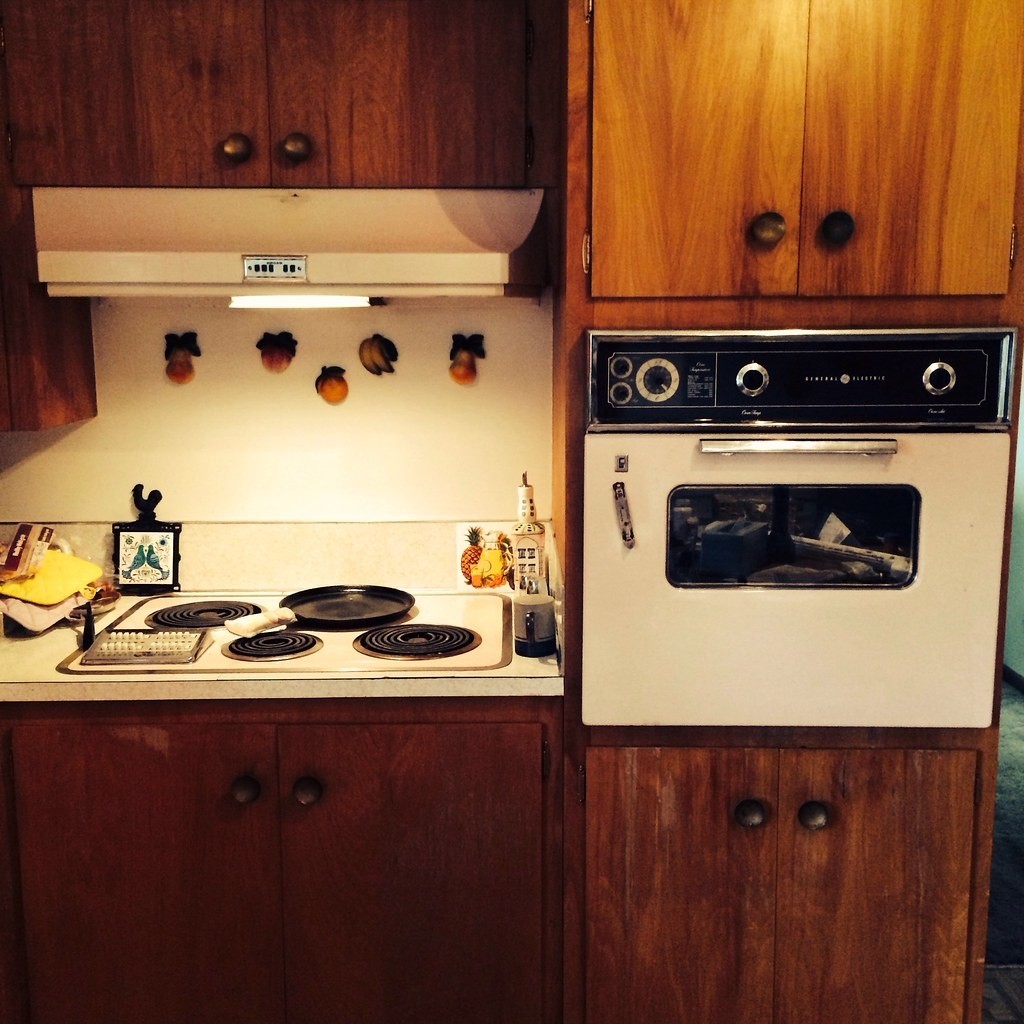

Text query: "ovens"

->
[581,327,1015,728]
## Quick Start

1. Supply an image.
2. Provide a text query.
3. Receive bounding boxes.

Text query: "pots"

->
[226,584,416,638]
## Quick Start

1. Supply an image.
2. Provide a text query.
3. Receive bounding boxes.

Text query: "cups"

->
[471,565,484,587]
[514,595,556,658]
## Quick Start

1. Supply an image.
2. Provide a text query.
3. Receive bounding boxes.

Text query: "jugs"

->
[478,542,513,588]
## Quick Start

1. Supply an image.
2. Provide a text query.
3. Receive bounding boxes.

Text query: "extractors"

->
[30,187,545,296]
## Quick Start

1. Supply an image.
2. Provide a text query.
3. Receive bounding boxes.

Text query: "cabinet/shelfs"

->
[0,0,563,192]
[523,0,1024,303]
[0,701,564,1024]
[562,729,998,1024]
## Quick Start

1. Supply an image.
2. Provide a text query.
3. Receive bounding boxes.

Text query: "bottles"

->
[512,471,548,594]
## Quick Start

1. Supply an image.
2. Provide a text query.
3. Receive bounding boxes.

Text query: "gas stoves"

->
[56,590,513,676]
